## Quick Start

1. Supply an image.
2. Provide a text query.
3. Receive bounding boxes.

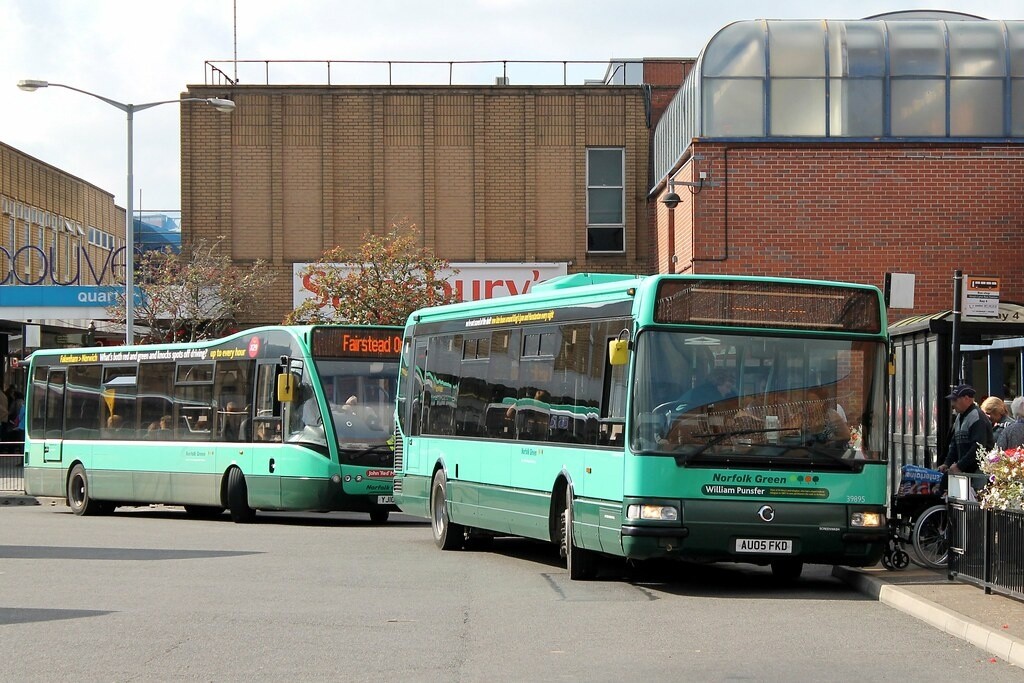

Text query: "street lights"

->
[14,79,237,344]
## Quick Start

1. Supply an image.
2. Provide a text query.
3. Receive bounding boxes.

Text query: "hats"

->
[945,384,976,399]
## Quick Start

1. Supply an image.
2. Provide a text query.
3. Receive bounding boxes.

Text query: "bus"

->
[394,272,898,584]
[23,324,407,525]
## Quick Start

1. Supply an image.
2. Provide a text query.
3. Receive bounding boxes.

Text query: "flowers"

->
[975,441,1024,512]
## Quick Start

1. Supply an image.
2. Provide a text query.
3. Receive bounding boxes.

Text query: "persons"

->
[938,384,1024,495]
[107,389,335,441]
[507,364,851,449]
[0,382,25,466]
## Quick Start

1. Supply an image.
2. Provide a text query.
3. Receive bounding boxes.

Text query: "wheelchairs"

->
[882,470,973,572]
